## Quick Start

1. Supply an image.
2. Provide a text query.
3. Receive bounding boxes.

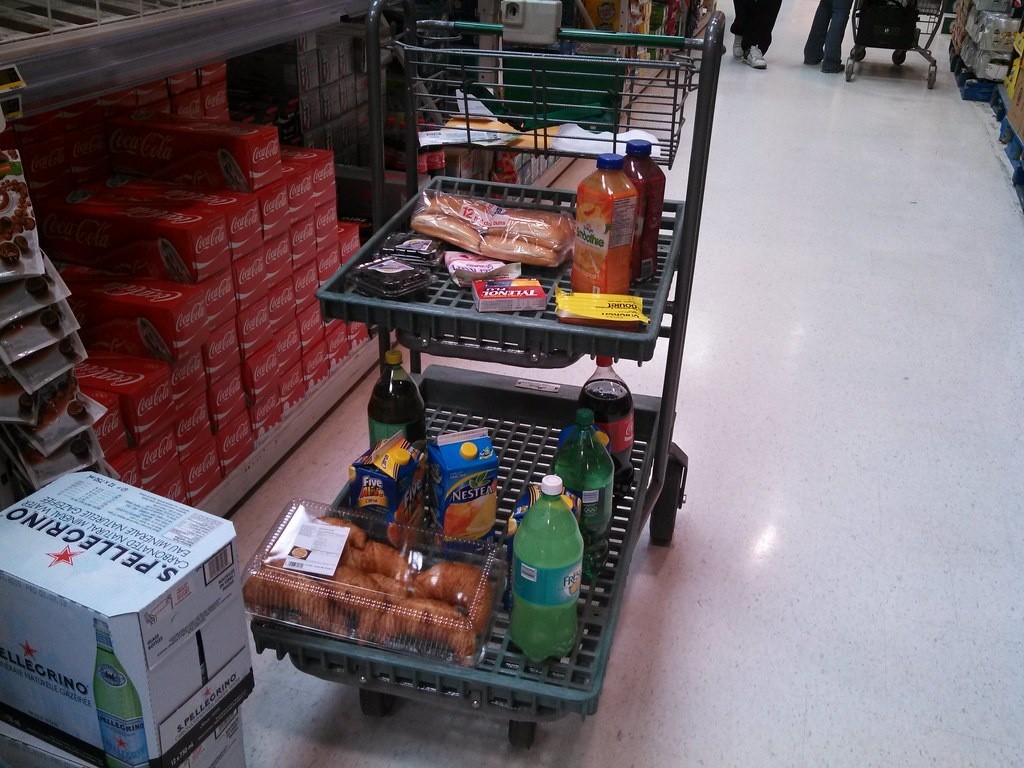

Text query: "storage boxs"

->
[584,0,680,93]
[0,24,390,768]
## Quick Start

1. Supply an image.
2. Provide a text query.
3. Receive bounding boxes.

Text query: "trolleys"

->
[249,0,726,751]
[845,0,946,89]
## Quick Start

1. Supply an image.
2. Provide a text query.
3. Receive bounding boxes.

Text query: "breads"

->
[411,195,577,266]
[243,516,494,662]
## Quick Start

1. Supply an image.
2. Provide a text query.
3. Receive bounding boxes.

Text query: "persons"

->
[803,0,853,73]
[730,0,782,69]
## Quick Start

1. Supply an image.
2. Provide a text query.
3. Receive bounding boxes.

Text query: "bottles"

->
[88,618,151,768]
[582,355,636,485]
[367,351,426,453]
[618,140,667,282]
[510,475,586,661]
[554,409,613,579]
[572,153,638,299]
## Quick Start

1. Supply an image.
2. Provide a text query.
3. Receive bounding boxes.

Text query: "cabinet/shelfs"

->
[0,0,503,501]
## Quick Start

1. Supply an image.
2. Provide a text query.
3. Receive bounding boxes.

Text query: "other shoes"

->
[828,64,845,72]
[743,44,767,68]
[733,35,744,58]
